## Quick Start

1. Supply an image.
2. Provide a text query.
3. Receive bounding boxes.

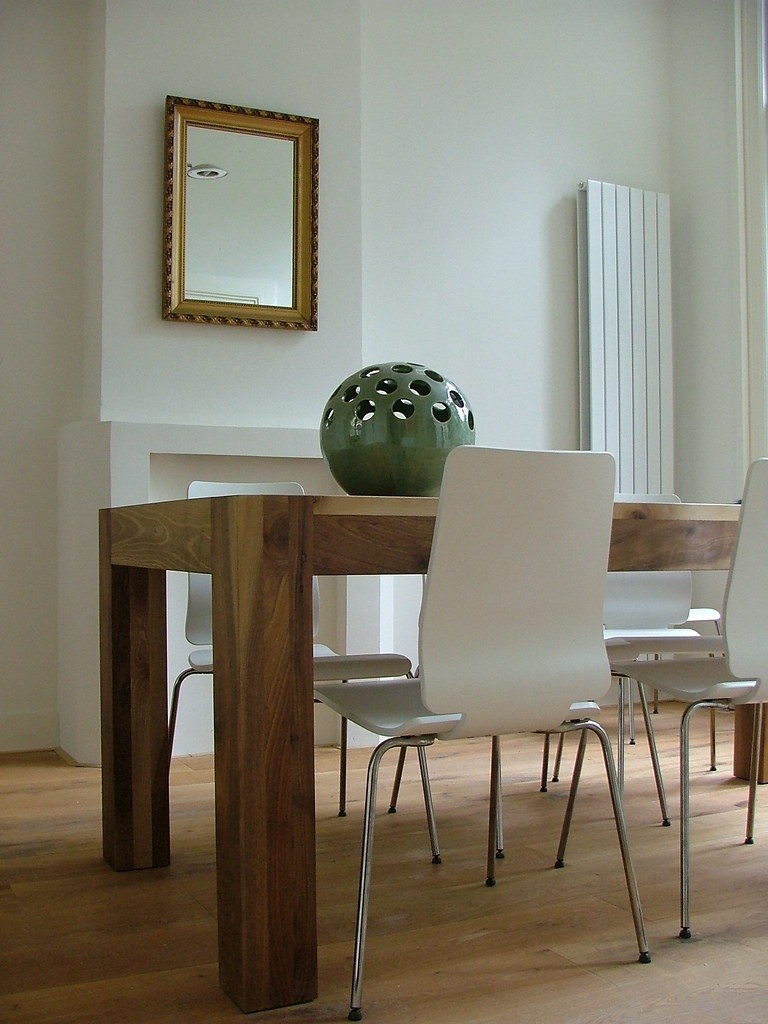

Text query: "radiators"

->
[572,175,676,497]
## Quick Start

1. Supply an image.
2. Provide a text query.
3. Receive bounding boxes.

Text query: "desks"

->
[95,486,740,1017]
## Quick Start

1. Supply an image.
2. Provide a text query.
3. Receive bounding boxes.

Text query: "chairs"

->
[165,493,726,868]
[552,454,768,942]
[311,441,654,1023]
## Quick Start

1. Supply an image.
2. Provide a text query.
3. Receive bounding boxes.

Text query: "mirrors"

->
[162,94,321,333]
